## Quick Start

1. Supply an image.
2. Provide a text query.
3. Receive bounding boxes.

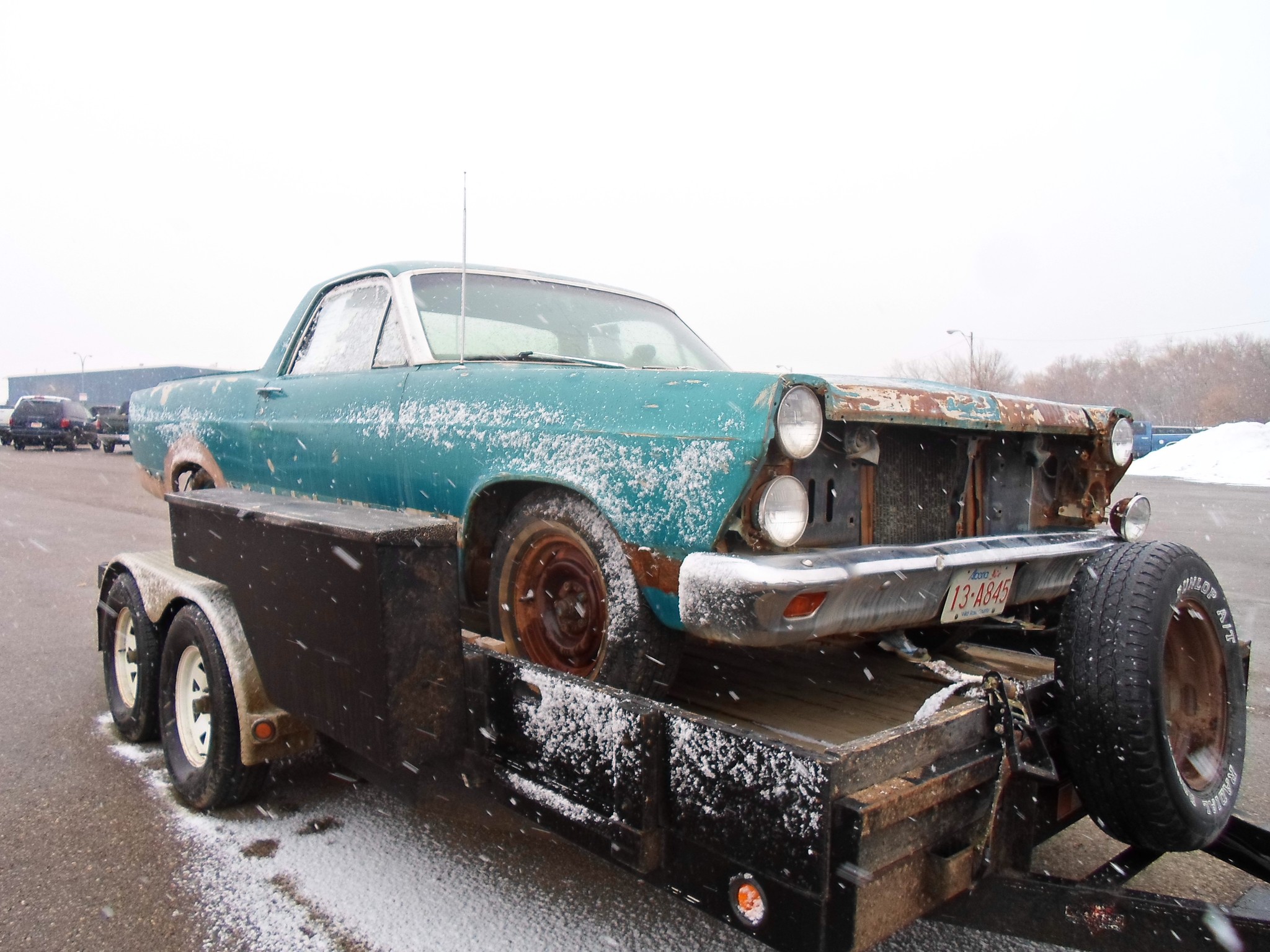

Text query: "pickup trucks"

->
[97,401,130,453]
[0,405,14,446]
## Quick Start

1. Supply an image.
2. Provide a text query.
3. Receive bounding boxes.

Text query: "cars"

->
[126,261,1144,683]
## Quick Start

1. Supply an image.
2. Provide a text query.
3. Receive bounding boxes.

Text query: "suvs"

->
[9,400,101,450]
[88,406,119,418]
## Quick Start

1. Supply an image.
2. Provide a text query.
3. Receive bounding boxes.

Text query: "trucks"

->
[1130,421,1209,460]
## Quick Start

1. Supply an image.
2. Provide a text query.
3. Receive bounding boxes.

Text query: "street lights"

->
[947,328,973,387]
[75,352,92,406]
